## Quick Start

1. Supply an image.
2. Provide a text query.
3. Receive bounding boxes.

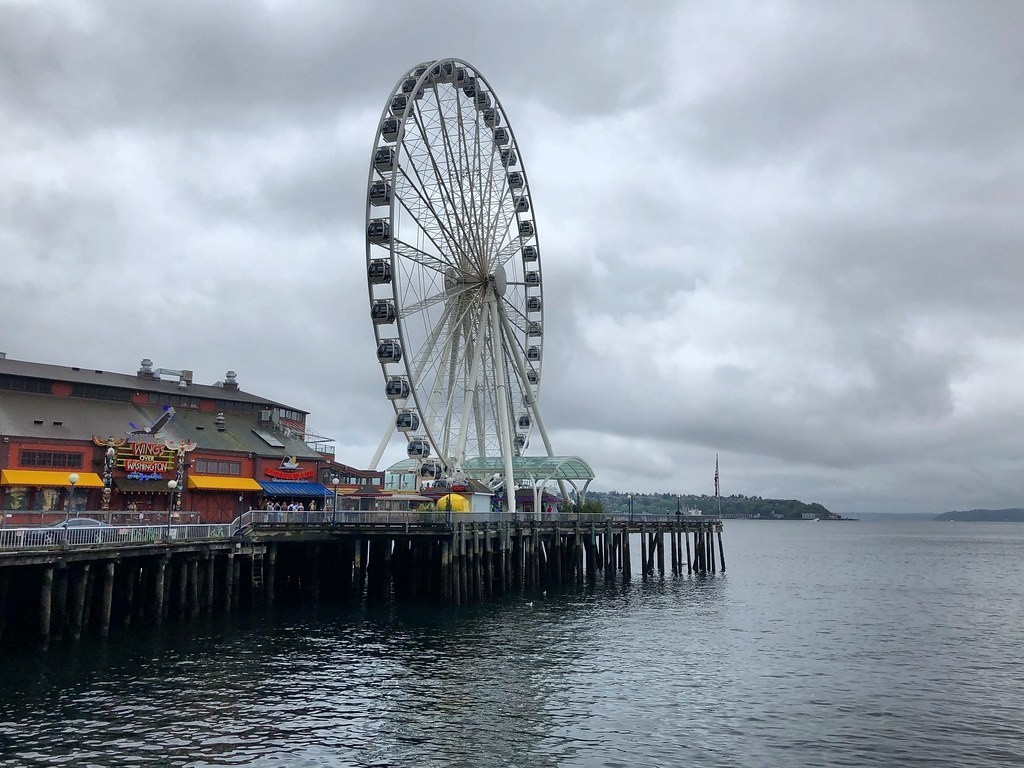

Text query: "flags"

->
[714,456,719,496]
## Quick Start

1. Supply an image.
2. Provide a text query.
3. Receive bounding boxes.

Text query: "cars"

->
[26,517,114,545]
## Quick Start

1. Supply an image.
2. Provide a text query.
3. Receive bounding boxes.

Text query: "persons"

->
[326,504,333,522]
[259,499,316,522]
[546,505,552,521]
[666,509,670,515]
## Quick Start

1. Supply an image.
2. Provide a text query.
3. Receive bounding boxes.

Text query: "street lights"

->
[513,485,520,528]
[676,495,681,522]
[576,488,582,528]
[445,477,454,533]
[59,474,80,551]
[160,479,178,545]
[627,495,631,528]
[332,477,340,529]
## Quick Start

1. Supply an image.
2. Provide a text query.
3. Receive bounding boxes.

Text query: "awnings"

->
[1,469,105,488]
[258,482,334,497]
[114,477,182,494]
[376,495,433,500]
[187,476,263,491]
[343,494,393,497]
